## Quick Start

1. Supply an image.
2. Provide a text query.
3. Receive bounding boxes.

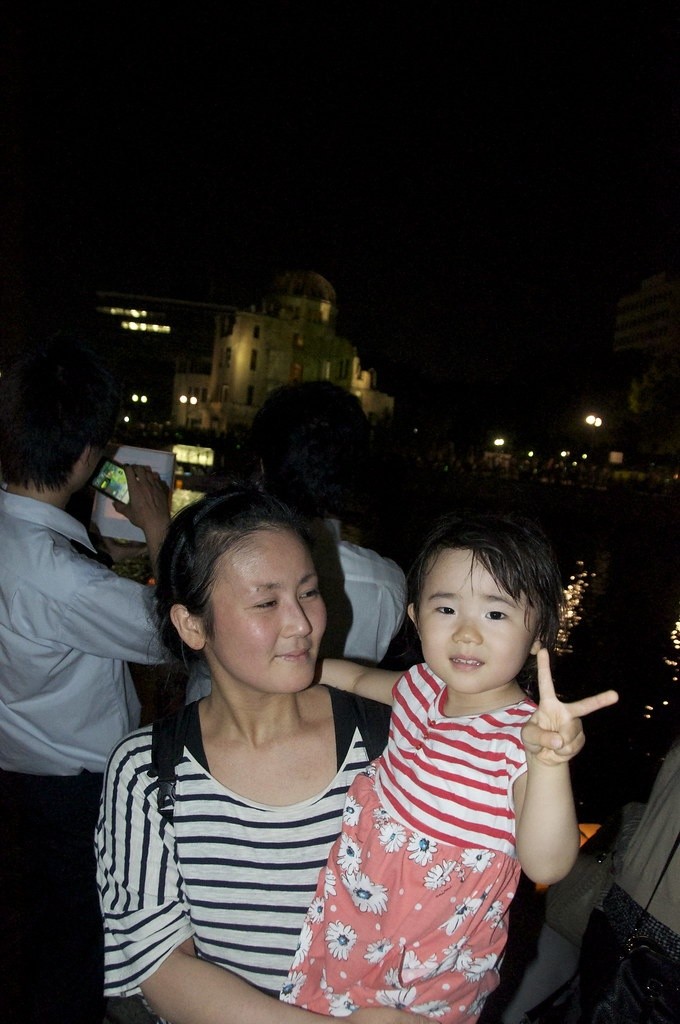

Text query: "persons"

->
[501,736,680,1024]
[184,380,407,703]
[281,520,618,1023]
[94,494,434,1022]
[0,363,175,1023]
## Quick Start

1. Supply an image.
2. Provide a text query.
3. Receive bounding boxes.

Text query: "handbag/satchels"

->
[583,936,680,1024]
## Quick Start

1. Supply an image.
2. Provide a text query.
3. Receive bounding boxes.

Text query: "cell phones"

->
[86,455,130,504]
